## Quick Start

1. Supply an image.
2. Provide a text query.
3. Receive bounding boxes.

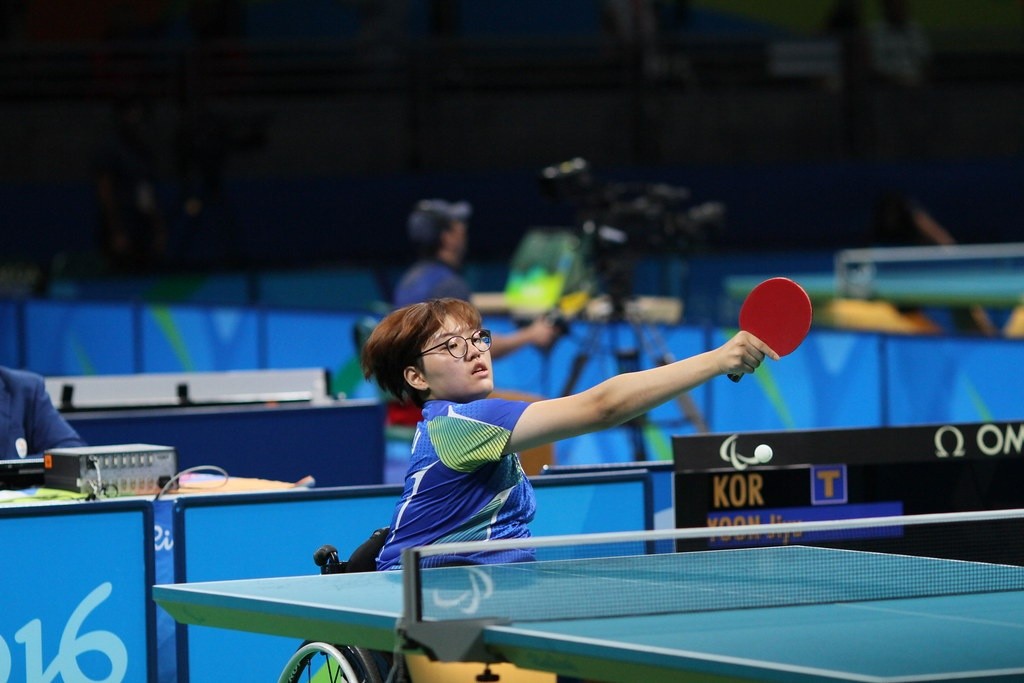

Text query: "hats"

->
[407,199,472,243]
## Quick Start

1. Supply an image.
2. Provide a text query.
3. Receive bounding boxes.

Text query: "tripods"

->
[536,264,709,462]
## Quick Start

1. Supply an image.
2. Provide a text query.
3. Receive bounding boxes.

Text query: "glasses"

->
[403,329,492,384]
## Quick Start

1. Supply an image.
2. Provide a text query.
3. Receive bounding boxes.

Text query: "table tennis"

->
[754,443,773,464]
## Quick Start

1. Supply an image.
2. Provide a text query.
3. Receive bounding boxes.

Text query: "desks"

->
[0,366,1024,683]
[725,270,1024,336]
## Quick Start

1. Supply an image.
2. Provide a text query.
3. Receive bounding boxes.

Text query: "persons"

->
[361,298,780,682]
[0,367,85,460]
[814,201,1024,341]
[97,177,202,277]
[395,201,555,360]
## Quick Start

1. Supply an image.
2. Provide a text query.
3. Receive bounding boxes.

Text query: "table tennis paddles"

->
[727,275,814,384]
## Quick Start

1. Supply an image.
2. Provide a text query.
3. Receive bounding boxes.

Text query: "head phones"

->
[414,201,454,231]
